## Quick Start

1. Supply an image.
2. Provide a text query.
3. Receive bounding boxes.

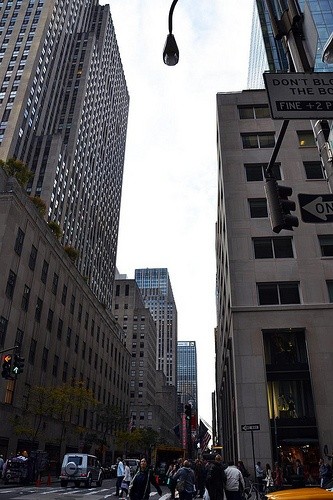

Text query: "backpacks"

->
[133,473,145,487]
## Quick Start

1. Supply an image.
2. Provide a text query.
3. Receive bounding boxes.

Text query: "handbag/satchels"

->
[121,483,128,491]
[178,480,194,495]
[238,478,244,492]
[203,488,210,500]
[167,478,170,484]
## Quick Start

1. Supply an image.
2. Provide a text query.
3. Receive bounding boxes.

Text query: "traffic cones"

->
[35,474,42,487]
[46,473,52,487]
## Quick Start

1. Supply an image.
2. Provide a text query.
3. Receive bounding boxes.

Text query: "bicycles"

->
[243,480,277,500]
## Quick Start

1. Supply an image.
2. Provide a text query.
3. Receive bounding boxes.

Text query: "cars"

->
[263,487,333,500]
[103,464,117,479]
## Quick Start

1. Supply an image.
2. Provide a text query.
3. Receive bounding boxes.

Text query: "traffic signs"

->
[240,424,261,432]
[297,193,333,224]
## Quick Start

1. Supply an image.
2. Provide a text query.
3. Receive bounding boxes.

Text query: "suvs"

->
[59,453,105,489]
[124,459,141,476]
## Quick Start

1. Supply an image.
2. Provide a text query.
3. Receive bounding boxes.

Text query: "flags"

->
[199,421,211,453]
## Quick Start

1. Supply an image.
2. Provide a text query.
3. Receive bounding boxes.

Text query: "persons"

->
[255,459,328,493]
[0,455,20,481]
[278,394,298,418]
[114,454,250,500]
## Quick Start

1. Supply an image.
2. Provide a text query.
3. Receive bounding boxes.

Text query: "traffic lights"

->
[12,353,25,374]
[2,354,12,379]
[264,180,299,233]
